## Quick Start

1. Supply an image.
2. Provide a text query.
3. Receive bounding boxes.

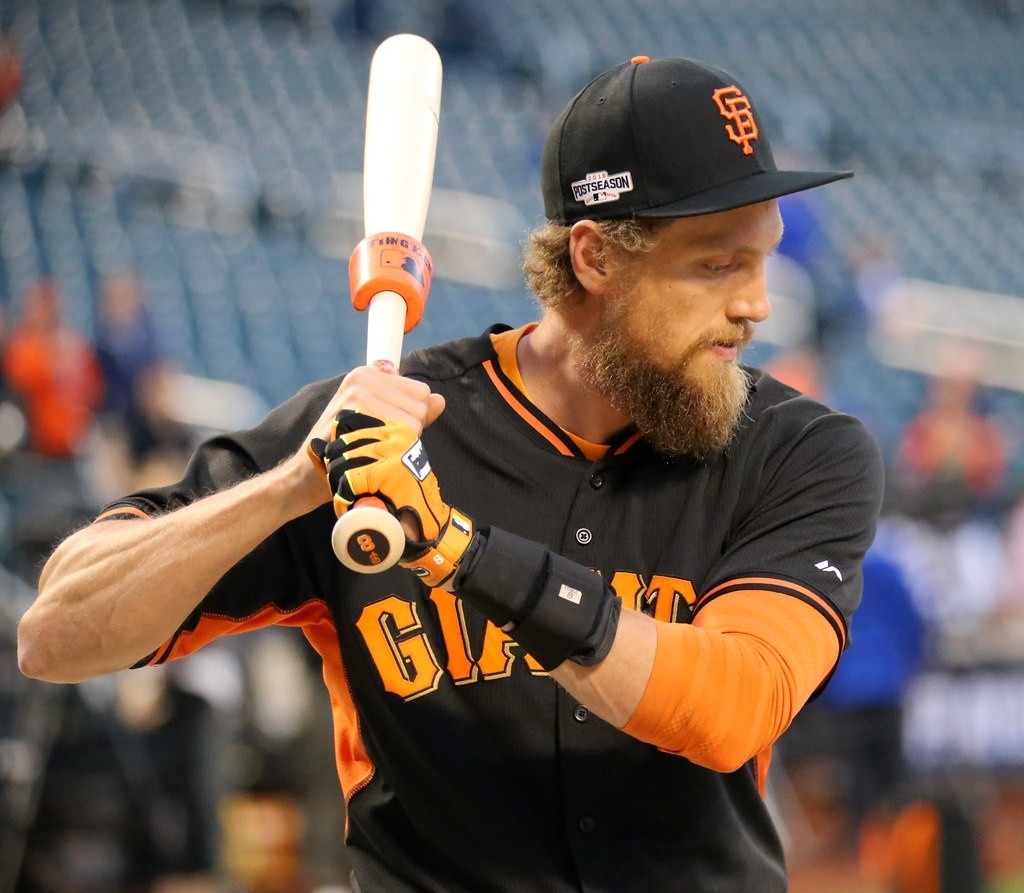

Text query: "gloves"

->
[305,408,475,588]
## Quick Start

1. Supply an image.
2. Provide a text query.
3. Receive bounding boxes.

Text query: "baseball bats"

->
[326,32,450,577]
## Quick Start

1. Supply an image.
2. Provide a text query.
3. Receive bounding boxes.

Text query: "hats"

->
[542,56,854,227]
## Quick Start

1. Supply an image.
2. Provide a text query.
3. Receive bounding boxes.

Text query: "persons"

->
[0,56,1024,893]
